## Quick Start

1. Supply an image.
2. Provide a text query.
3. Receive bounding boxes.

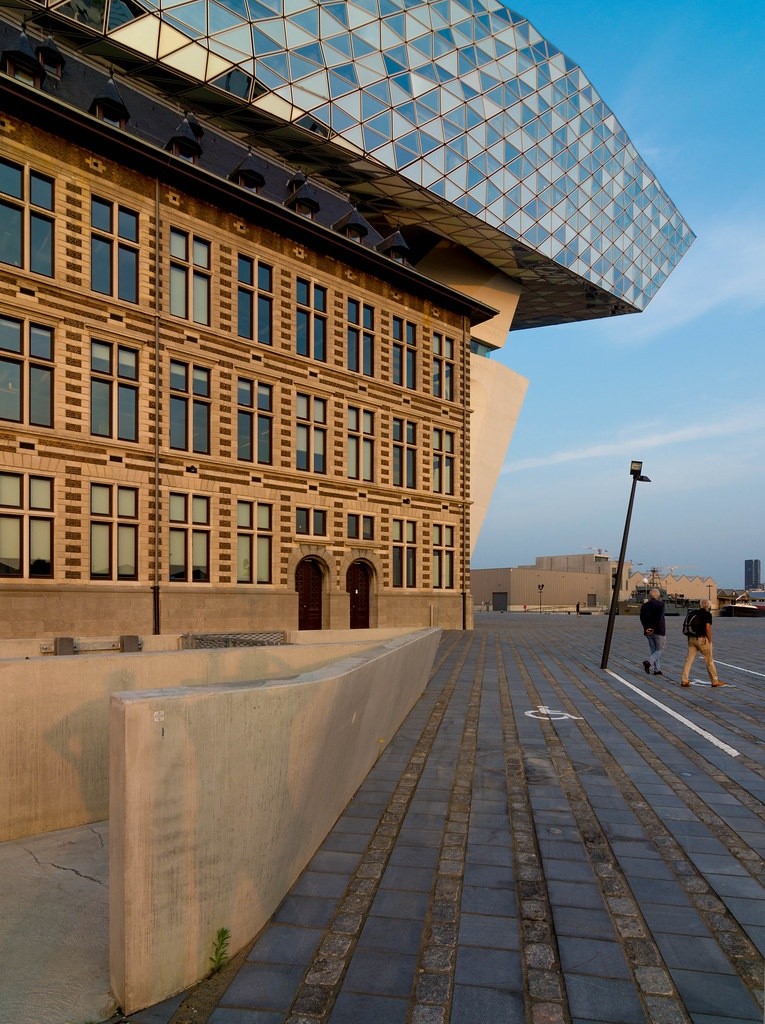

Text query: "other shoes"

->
[654,670,663,675]
[643,661,652,674]
[712,679,726,687]
[682,681,690,687]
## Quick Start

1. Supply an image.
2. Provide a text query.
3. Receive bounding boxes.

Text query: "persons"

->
[640,588,666,675]
[681,599,727,688]
[576,602,580,615]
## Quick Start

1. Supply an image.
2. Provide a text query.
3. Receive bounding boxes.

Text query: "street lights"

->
[600,461,652,669]
[537,584,544,613]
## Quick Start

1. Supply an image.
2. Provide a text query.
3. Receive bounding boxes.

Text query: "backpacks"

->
[682,610,706,635]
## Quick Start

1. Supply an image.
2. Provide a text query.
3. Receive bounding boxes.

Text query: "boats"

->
[720,598,759,616]
[606,565,702,616]
